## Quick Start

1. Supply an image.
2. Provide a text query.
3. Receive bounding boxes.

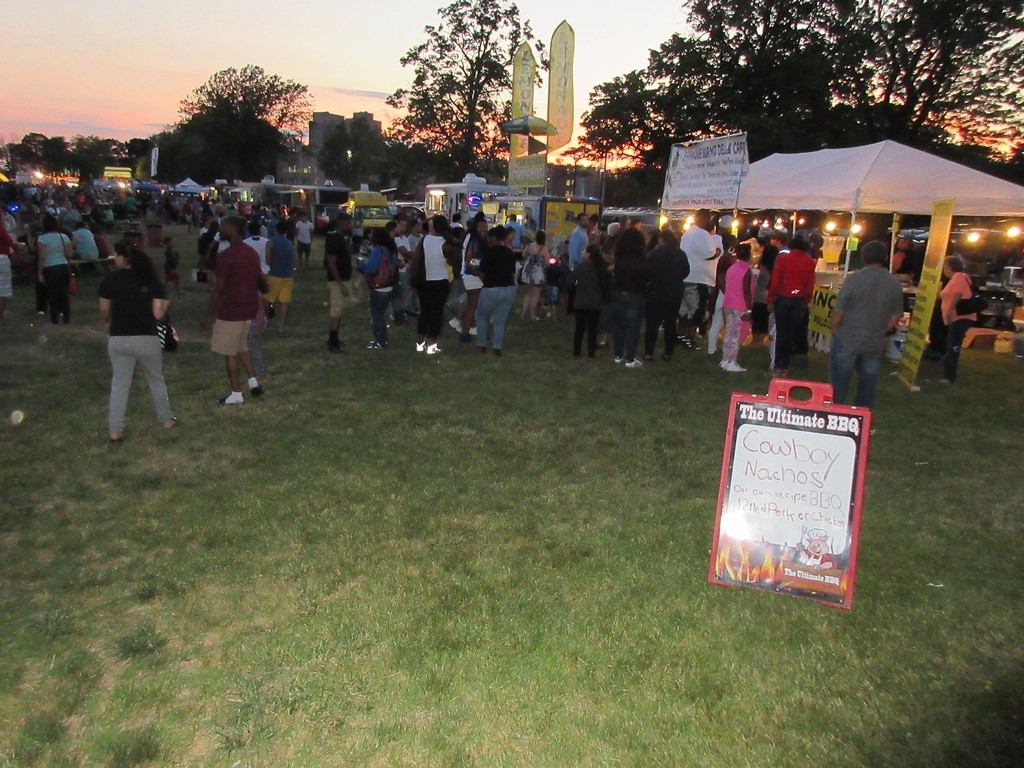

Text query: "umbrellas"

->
[501,114,558,197]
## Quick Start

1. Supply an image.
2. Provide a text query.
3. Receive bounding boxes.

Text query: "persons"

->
[474,224,517,357]
[97,240,180,446]
[766,228,815,377]
[936,255,977,385]
[37,215,73,326]
[829,239,903,410]
[565,244,612,361]
[0,180,977,410]
[255,222,297,337]
[323,212,355,353]
[355,228,401,349]
[719,242,749,373]
[410,214,461,355]
[198,216,265,407]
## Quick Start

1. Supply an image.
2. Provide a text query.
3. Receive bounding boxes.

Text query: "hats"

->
[719,214,733,229]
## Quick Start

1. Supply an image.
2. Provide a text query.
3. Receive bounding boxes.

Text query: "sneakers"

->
[614,356,623,363]
[624,358,642,368]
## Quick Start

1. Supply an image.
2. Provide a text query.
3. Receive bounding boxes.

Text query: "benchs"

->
[68,258,115,277]
[81,213,140,231]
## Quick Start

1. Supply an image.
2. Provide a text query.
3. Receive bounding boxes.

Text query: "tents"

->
[168,177,210,194]
[737,139,1024,279]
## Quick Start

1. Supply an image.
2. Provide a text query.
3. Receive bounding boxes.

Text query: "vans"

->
[347,191,393,245]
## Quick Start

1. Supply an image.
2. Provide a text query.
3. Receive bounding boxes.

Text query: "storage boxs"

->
[961,327,997,351]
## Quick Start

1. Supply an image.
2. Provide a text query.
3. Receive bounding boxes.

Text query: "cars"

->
[899,227,1007,246]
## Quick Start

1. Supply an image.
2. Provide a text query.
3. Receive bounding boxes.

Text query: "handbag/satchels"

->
[156,309,177,352]
[364,246,397,290]
[68,273,77,293]
[517,267,529,286]
[956,277,988,315]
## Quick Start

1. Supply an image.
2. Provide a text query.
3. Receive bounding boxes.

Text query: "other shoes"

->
[493,349,502,356]
[327,338,346,353]
[217,395,246,405]
[449,318,463,333]
[389,308,420,326]
[767,368,790,377]
[677,334,701,351]
[365,339,389,350]
[250,384,264,396]
[719,359,748,371]
[163,416,179,428]
[415,341,443,354]
[468,327,477,335]
[469,345,486,354]
[109,425,128,445]
